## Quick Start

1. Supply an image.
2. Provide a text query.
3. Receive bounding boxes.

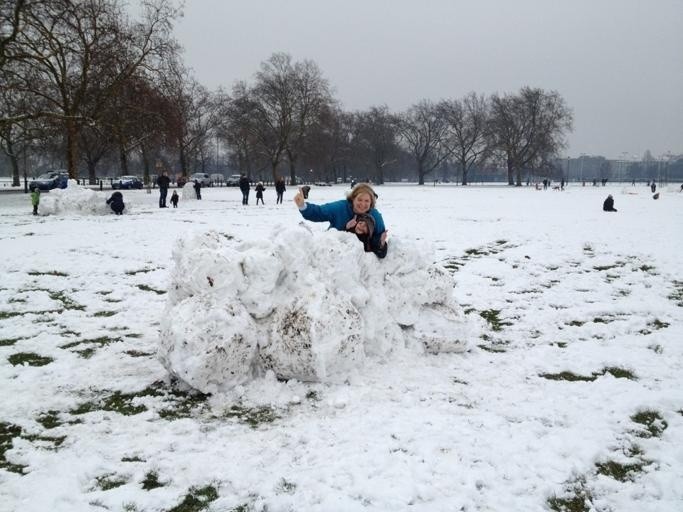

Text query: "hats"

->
[357,214,375,239]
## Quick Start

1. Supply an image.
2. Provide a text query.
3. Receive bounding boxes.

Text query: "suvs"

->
[29,172,80,192]
[226,174,241,187]
[187,173,212,188]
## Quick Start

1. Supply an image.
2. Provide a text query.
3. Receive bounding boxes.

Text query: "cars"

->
[112,176,143,189]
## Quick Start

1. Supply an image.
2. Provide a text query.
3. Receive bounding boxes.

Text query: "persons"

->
[254,181,266,206]
[238,172,250,206]
[274,176,286,205]
[156,169,171,209]
[293,182,388,260]
[192,178,201,200]
[533,176,682,199]
[169,190,179,209]
[143,175,151,194]
[105,191,125,215]
[297,185,311,199]
[602,194,617,212]
[342,212,376,254]
[29,188,40,216]
